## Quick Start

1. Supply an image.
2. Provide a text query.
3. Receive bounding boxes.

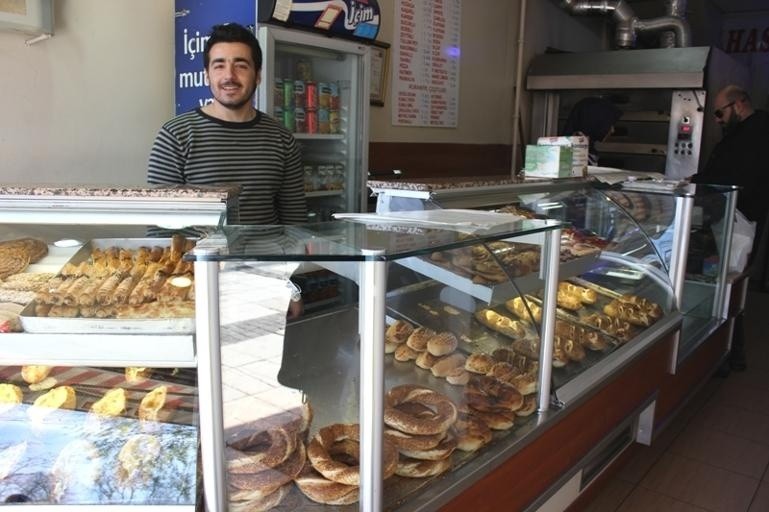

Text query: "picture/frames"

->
[367,37,391,107]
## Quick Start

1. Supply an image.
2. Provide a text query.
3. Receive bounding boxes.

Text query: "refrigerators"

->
[173,0,382,317]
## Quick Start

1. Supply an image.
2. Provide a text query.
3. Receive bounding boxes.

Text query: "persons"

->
[679,84,769,377]
[146,21,313,321]
[566,98,624,166]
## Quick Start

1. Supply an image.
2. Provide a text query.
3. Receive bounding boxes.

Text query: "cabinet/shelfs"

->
[599,176,742,371]
[184,219,572,511]
[3,196,228,505]
[365,178,677,395]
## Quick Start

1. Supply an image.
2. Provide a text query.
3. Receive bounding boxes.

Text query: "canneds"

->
[274,75,341,135]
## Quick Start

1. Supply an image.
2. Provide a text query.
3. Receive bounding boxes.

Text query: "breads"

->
[1,365,177,504]
[427,207,603,282]
[0,237,195,333]
[223,283,663,511]
[602,189,676,257]
